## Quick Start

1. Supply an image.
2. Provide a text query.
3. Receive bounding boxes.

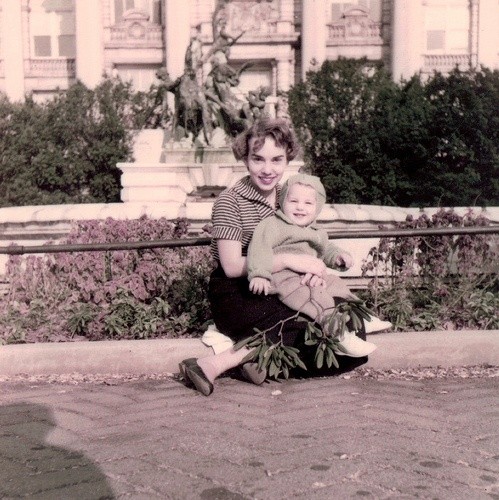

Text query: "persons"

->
[179,115,368,396]
[144,0,272,147]
[245,174,392,357]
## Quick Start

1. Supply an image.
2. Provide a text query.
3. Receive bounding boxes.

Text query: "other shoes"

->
[362,317,392,334]
[335,333,379,357]
[179,357,214,396]
[221,362,268,386]
